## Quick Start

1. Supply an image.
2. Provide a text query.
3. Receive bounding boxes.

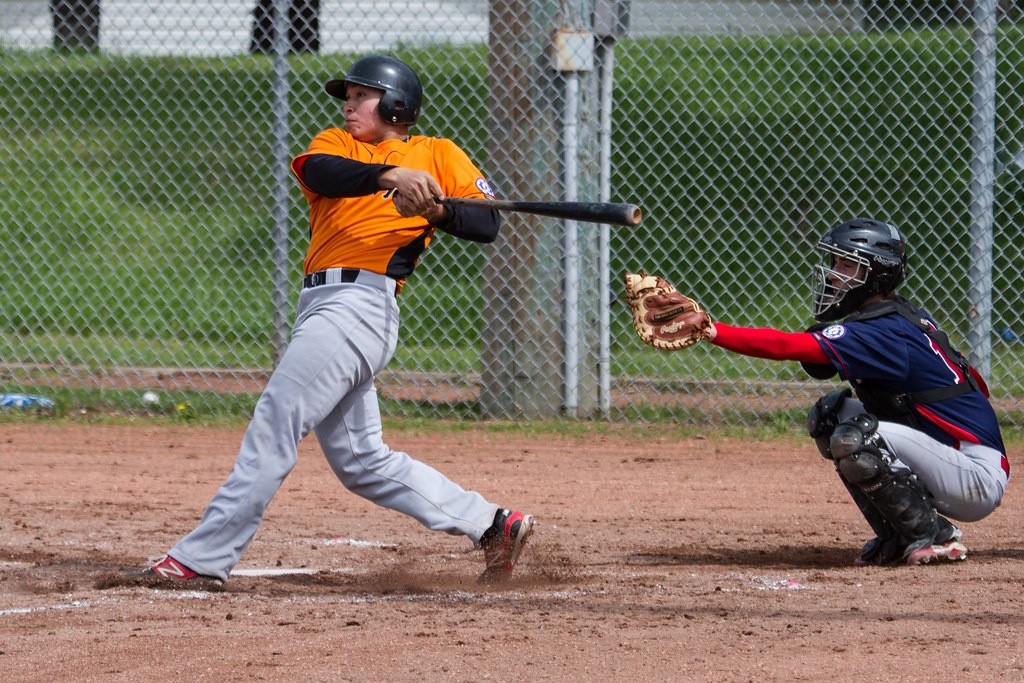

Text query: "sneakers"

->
[856,531,969,566]
[123,555,224,588]
[477,510,535,584]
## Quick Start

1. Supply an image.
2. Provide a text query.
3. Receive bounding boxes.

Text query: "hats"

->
[325,56,423,126]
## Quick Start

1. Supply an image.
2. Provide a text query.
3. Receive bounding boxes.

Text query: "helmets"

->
[812,218,912,322]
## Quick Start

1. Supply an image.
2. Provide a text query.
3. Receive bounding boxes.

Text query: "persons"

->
[625,220,1010,567]
[136,56,535,588]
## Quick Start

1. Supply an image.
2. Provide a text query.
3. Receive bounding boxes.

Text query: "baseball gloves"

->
[624,272,712,349]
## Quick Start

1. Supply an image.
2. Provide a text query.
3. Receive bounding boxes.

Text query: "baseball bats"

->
[393,188,642,226]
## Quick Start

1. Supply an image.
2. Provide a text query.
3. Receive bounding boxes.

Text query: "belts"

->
[304,269,359,288]
[953,440,1010,480]
